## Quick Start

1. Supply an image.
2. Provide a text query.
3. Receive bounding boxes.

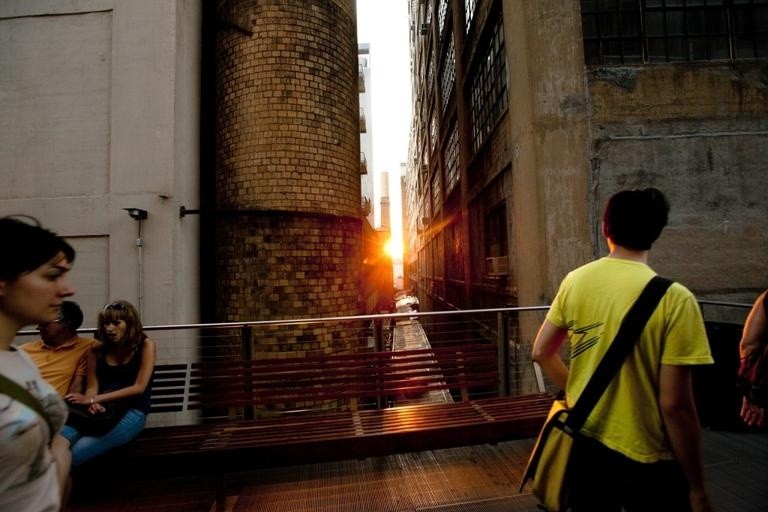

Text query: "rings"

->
[751,413,757,418]
[746,410,751,415]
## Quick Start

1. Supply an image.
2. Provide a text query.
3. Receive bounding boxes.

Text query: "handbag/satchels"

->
[518,397,576,511]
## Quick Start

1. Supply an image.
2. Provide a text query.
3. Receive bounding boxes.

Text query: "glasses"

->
[102,303,128,311]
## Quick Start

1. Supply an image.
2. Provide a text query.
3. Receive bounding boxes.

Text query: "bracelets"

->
[89,393,94,405]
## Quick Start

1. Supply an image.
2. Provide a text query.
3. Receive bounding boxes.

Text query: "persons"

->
[530,187,716,509]
[736,287,767,427]
[0,213,76,510]
[51,299,155,510]
[17,301,102,405]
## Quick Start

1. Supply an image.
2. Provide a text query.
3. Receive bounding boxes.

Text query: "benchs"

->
[129,336,562,486]
